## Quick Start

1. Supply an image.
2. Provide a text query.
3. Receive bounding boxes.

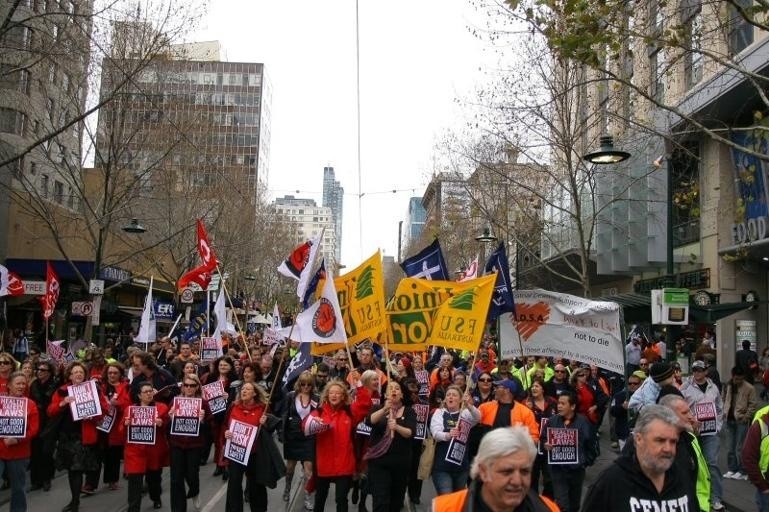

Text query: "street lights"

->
[579,109,686,368]
[92,214,148,346]
[265,283,291,321]
[225,265,258,281]
[476,219,520,292]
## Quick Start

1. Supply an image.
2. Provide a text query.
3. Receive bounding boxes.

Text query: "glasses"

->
[36,368,48,371]
[555,371,565,373]
[184,384,198,388]
[318,375,328,378]
[340,359,348,361]
[479,378,493,382]
[301,383,312,386]
[0,361,10,365]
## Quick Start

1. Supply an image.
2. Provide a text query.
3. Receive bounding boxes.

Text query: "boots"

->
[304,478,313,509]
[283,479,291,501]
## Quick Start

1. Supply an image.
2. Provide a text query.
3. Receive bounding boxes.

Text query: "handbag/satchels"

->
[417,438,437,481]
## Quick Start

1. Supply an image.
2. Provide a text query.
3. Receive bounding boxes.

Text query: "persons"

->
[0,322,768,511]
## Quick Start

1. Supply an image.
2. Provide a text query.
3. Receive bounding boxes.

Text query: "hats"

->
[649,362,674,383]
[692,360,705,369]
[492,380,517,393]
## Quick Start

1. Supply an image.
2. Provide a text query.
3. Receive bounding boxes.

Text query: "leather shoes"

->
[62,500,79,511]
[213,466,223,476]
[223,472,228,480]
[153,498,161,509]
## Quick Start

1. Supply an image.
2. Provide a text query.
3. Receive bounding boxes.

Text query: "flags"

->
[176,219,219,296]
[37,260,61,320]
[0,264,24,298]
[212,225,625,374]
[131,275,159,343]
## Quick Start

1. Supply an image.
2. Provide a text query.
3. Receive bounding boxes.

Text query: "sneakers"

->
[723,471,735,478]
[110,482,118,489]
[192,495,201,509]
[732,472,747,479]
[713,503,725,511]
[81,484,98,493]
